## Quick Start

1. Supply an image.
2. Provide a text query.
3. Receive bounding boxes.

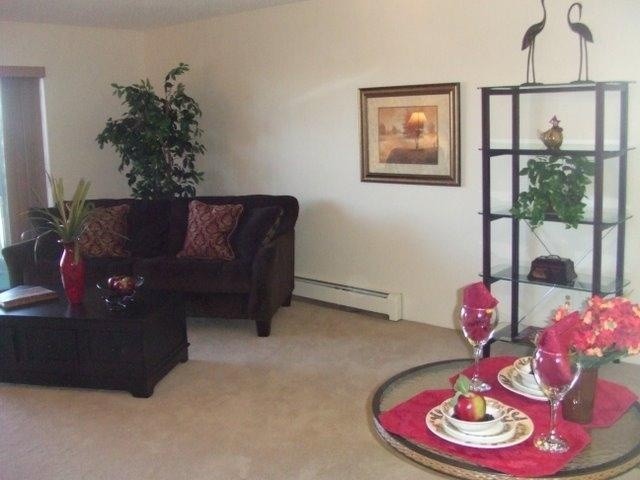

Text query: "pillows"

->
[175,200,244,262]
[78,203,132,260]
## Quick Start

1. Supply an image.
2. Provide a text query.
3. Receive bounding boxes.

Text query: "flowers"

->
[550,293,640,372]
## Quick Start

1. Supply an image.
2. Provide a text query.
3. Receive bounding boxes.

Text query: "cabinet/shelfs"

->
[478,81,628,348]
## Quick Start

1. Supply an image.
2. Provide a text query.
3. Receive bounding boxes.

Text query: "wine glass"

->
[459,305,496,392]
[533,346,581,453]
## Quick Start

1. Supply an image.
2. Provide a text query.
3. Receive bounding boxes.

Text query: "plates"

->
[498,366,550,402]
[426,406,535,449]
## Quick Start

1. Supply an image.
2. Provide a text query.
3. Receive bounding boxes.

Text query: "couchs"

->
[2,195,299,337]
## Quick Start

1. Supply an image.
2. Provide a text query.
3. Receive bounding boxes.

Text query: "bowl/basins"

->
[514,356,544,385]
[438,396,507,431]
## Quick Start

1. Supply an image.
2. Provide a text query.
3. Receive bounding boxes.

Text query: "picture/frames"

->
[359,83,462,188]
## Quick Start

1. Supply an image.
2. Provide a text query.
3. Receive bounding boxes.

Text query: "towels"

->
[449,356,640,429]
[537,310,589,388]
[462,280,498,341]
[379,390,592,478]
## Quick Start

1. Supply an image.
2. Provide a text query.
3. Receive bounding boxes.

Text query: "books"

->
[0,285,57,308]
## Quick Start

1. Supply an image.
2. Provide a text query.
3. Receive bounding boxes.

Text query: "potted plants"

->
[18,170,93,307]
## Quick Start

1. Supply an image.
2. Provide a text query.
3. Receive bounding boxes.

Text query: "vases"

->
[561,356,598,424]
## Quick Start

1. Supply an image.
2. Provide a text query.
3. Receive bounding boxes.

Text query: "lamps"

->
[407,111,427,151]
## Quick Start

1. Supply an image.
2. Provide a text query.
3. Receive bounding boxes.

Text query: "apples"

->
[107,276,134,289]
[454,392,486,421]
